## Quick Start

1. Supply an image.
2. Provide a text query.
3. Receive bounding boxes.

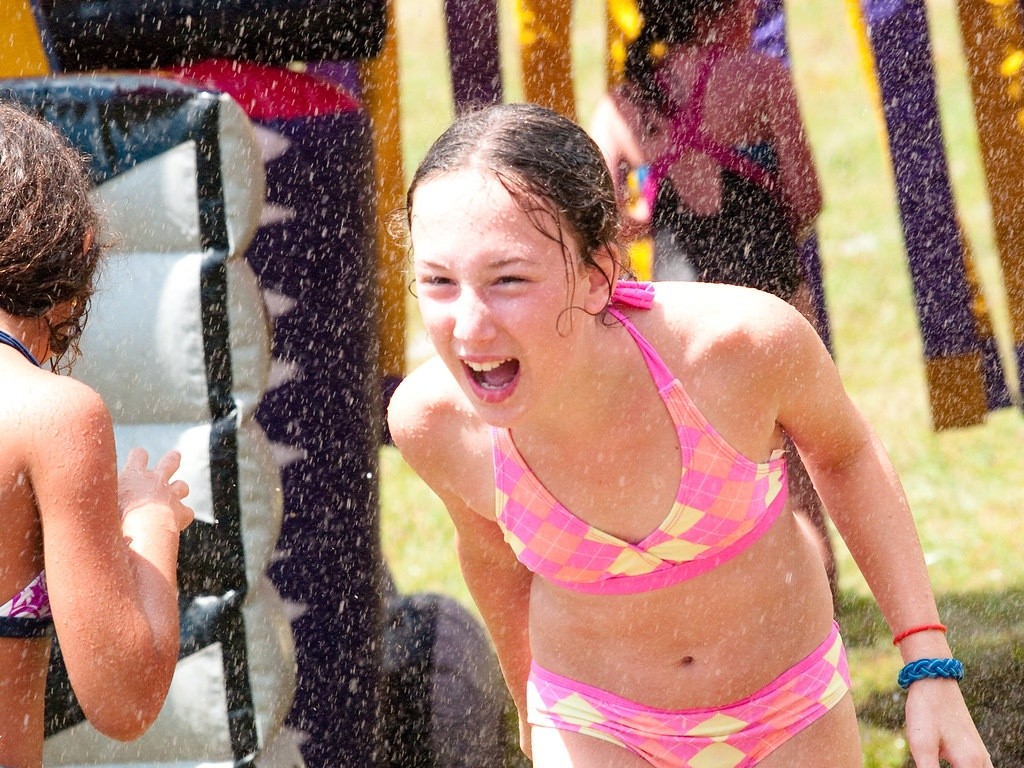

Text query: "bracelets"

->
[896,655,971,694]
[888,623,955,646]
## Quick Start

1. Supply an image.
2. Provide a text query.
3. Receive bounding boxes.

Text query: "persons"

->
[0,95,197,768]
[608,0,849,611]
[384,96,1000,768]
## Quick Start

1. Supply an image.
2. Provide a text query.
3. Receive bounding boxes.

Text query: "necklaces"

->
[0,325,44,373]
